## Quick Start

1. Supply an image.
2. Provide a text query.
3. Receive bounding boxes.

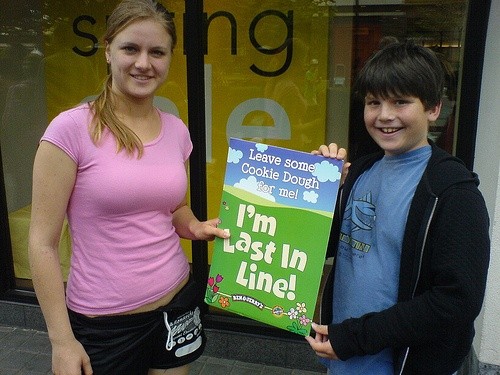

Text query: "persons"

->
[306,38,490,375]
[26,0,231,375]
